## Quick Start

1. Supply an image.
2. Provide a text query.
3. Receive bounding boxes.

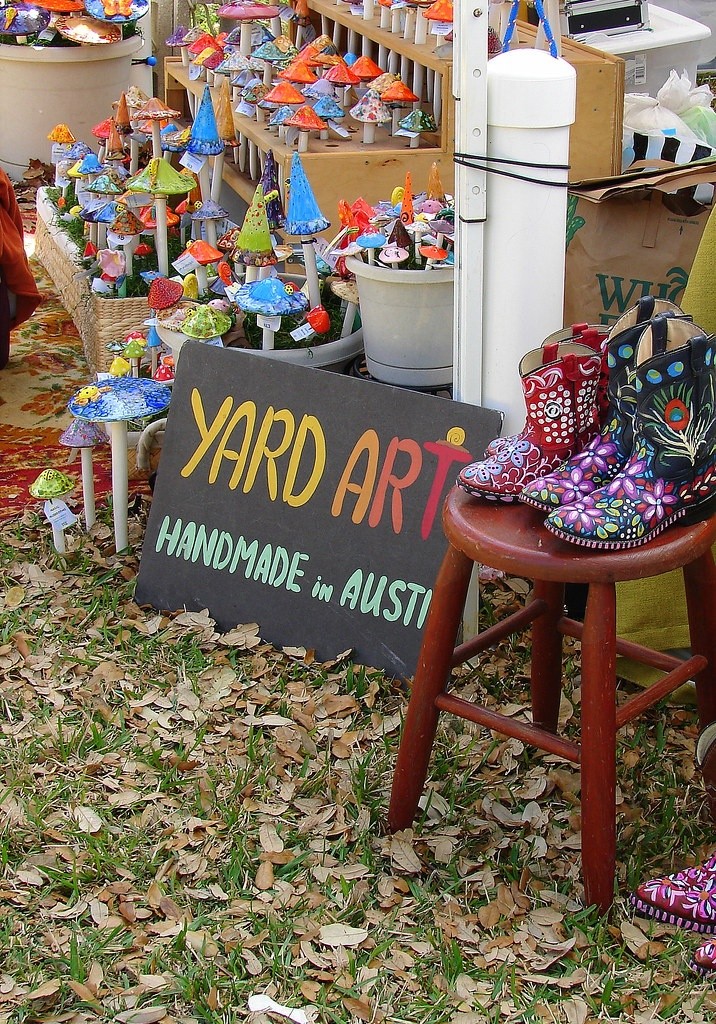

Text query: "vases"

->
[154,275,364,376]
[0,35,144,182]
[344,255,455,386]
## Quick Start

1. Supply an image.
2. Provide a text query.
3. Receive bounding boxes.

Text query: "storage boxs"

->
[566,2,711,99]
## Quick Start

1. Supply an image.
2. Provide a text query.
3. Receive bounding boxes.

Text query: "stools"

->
[379,484,716,916]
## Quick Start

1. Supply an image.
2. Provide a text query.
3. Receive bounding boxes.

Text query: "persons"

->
[0,167,42,370]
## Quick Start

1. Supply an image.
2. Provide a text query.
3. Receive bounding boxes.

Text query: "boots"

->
[455,323,611,502]
[628,850,716,970]
[519,295,716,550]
